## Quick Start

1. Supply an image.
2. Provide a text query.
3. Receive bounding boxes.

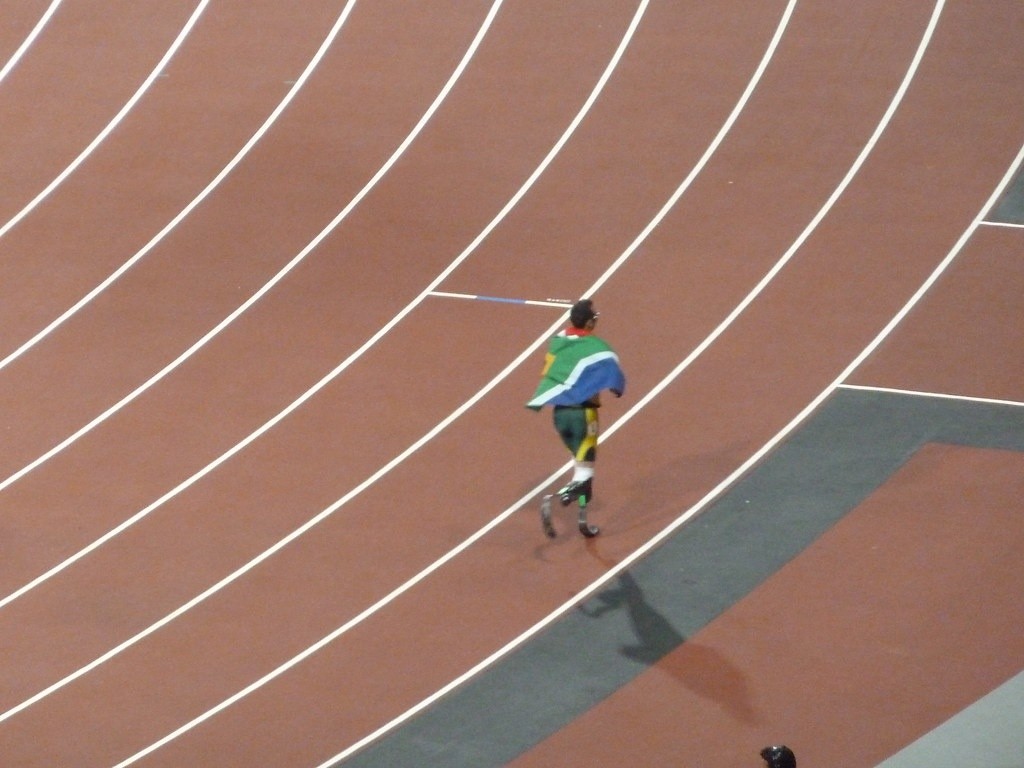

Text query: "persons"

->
[534,300,600,540]
[760,746,798,768]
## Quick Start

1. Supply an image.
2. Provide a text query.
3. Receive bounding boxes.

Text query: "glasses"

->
[590,312,600,322]
